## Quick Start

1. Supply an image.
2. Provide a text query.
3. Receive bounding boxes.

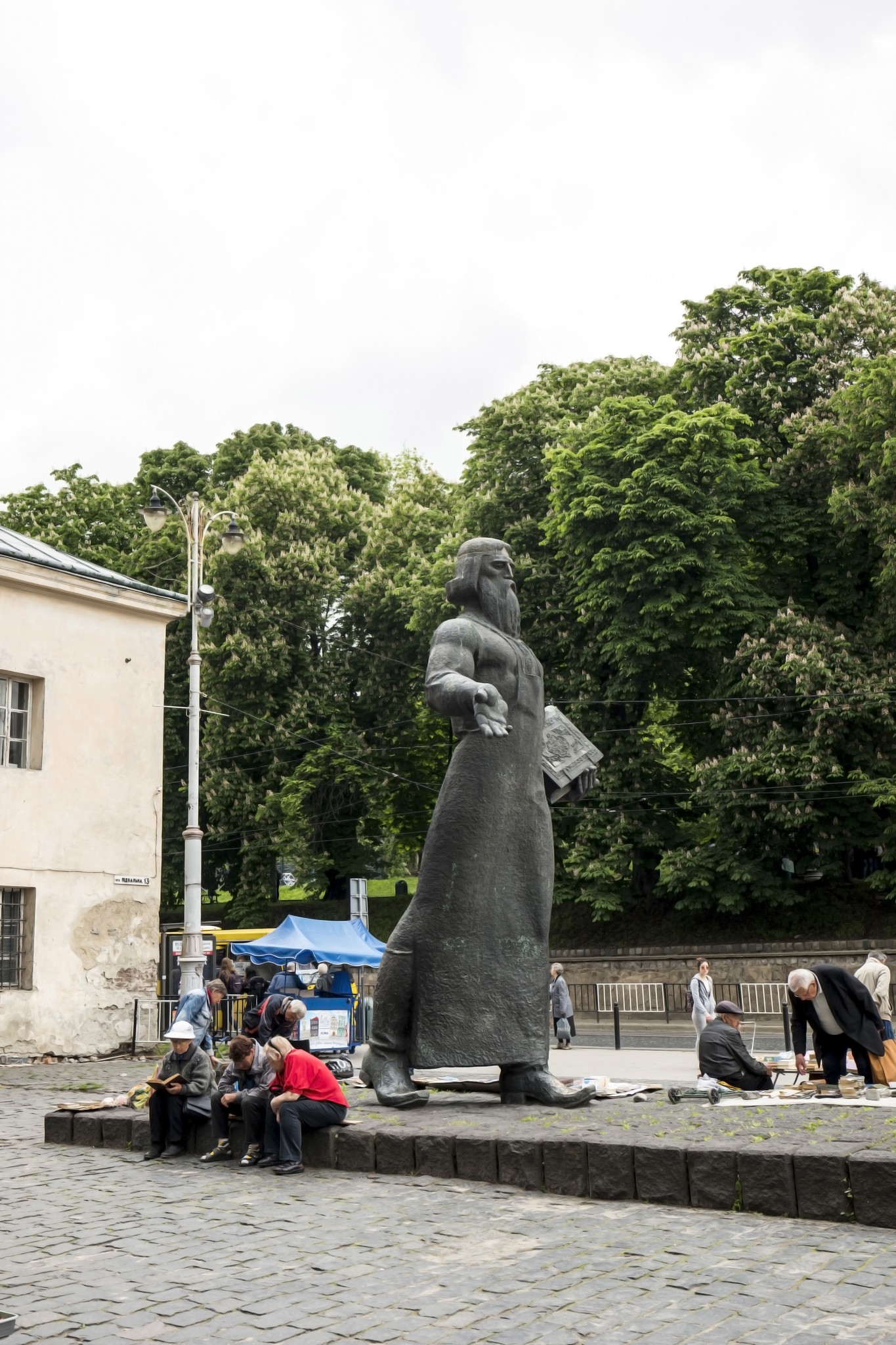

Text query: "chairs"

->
[288,1039,311,1054]
[211,1036,230,1061]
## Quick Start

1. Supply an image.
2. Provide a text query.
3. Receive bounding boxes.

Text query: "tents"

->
[231,916,385,969]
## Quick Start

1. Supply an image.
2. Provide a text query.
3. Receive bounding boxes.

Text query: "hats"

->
[713,1001,744,1015]
[163,1020,196,1039]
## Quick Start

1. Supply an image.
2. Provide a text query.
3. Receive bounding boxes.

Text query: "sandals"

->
[238,1152,266,1166]
[199,1148,234,1163]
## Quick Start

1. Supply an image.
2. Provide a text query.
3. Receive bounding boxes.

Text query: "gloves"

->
[561,1013,566,1018]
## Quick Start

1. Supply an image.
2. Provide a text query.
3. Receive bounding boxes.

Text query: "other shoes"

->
[257,1154,285,1167]
[161,1145,186,1158]
[272,1160,304,1175]
[144,1146,166,1159]
[231,1019,237,1030]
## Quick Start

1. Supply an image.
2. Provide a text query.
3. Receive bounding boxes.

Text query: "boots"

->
[562,1042,572,1050]
[551,1042,565,1049]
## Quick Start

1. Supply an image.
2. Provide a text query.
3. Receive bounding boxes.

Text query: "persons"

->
[787,964,885,1085]
[686,957,716,1069]
[242,967,269,1019]
[698,1000,774,1091]
[853,950,895,1041]
[313,964,333,997]
[287,961,295,973]
[360,536,596,1112]
[200,1034,278,1166]
[549,963,576,1050]
[144,1021,217,1159]
[165,979,228,1067]
[219,958,239,1031]
[256,1035,349,1173]
[240,994,307,1047]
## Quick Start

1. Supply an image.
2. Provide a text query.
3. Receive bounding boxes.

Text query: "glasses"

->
[268,1037,283,1057]
[794,985,810,997]
[700,966,710,970]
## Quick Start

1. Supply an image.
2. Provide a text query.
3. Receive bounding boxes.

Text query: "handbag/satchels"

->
[556,1018,572,1039]
[867,1028,896,1087]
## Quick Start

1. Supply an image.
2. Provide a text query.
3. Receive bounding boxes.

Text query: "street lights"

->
[141,483,245,1002]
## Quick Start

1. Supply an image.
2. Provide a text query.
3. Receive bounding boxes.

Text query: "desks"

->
[772,1069,824,1087]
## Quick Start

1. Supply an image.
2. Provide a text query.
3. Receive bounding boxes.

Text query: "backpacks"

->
[229,974,242,994]
[685,979,701,1013]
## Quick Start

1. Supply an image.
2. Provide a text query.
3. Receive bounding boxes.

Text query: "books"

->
[778,1072,896,1099]
[146,1073,191,1092]
[763,1054,821,1071]
